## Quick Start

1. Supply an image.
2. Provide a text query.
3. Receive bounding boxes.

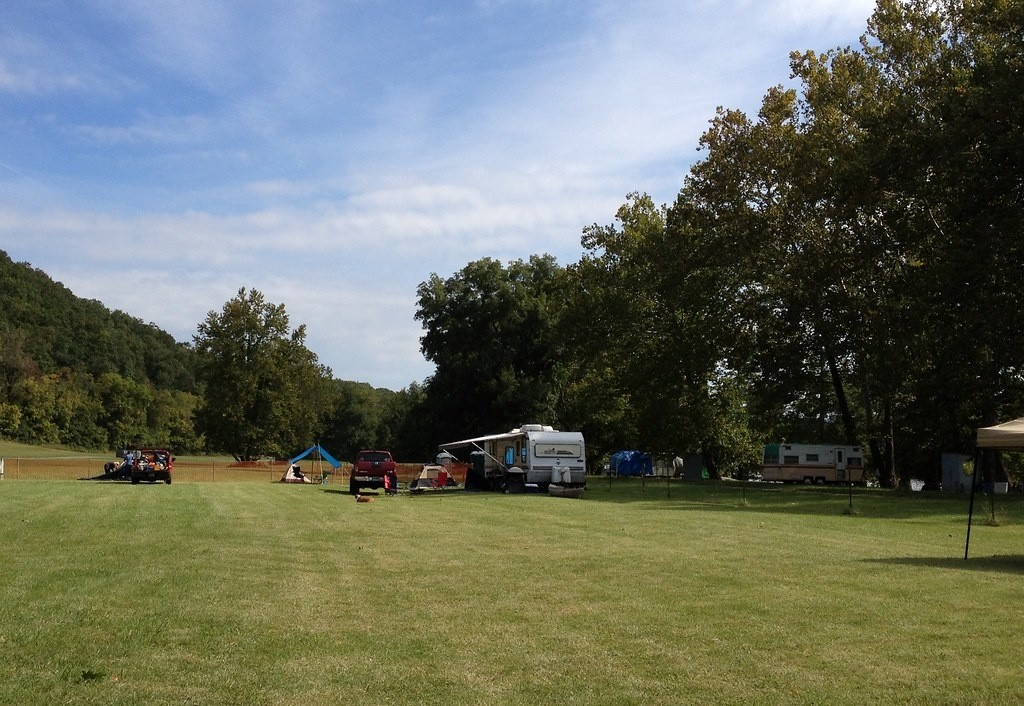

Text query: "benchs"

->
[313,477,321,485]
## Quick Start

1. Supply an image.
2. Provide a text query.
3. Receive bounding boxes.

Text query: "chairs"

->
[292,466,305,483]
[383,475,404,497]
[429,473,448,493]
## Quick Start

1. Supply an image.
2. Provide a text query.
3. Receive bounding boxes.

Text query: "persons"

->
[104,462,119,474]
[124,450,133,481]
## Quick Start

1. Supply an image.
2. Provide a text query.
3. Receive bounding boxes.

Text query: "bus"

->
[760,441,866,485]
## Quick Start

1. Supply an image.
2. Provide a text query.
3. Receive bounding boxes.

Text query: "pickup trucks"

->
[350,449,398,495]
[129,449,176,485]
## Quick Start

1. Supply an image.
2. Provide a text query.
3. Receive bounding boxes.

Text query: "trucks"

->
[438,424,588,493]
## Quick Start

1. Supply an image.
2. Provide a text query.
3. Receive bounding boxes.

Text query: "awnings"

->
[438,432,524,474]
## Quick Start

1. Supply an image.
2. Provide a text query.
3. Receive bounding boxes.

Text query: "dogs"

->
[355,494,375,503]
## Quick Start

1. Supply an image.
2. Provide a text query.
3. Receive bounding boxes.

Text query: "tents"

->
[281,464,312,483]
[610,450,683,477]
[963,412,1024,560]
